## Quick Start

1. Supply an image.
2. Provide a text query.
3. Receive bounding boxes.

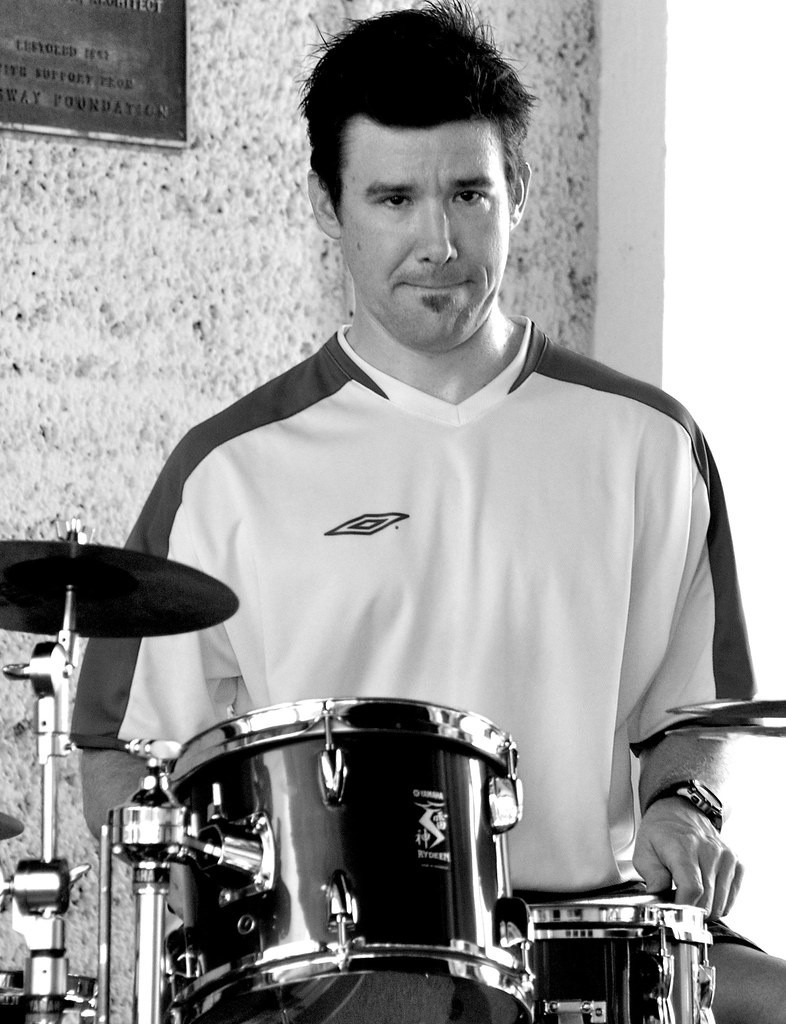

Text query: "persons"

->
[70,0,786,1024]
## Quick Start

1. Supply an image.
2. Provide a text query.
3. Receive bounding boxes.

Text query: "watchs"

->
[639,777,723,834]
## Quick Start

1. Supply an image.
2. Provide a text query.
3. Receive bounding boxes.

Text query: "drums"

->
[145,693,538,1024]
[518,894,717,1024]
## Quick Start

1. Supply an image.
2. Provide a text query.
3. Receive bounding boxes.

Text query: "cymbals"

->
[0,538,240,640]
[664,697,786,740]
